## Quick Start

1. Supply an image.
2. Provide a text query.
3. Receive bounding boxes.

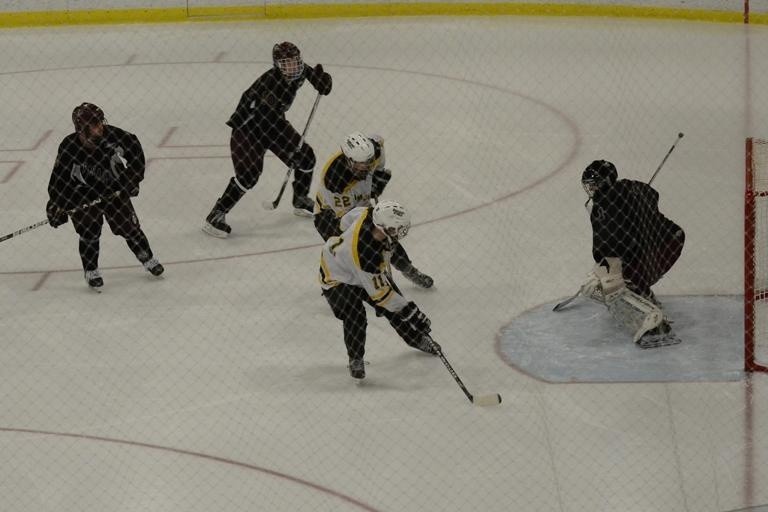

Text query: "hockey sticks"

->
[552,131,685,312]
[382,268,502,408]
[260,91,323,211]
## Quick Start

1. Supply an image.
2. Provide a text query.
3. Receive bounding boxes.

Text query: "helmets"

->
[341,131,376,172]
[71,102,107,141]
[272,41,304,82]
[372,200,411,245]
[581,160,619,199]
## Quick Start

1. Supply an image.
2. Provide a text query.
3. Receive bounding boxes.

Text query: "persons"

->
[46,102,164,293]
[313,132,433,288]
[317,201,441,386]
[581,160,685,348]
[202,42,332,239]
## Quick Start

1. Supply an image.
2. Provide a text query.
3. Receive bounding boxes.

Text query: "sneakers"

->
[348,355,366,380]
[633,311,672,343]
[204,198,235,233]
[143,256,164,276]
[413,334,441,355]
[403,264,434,288]
[292,181,315,214]
[84,269,104,288]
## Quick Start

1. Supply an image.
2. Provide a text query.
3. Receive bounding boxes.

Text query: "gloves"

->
[397,301,432,332]
[313,62,332,95]
[288,147,308,170]
[45,200,68,228]
